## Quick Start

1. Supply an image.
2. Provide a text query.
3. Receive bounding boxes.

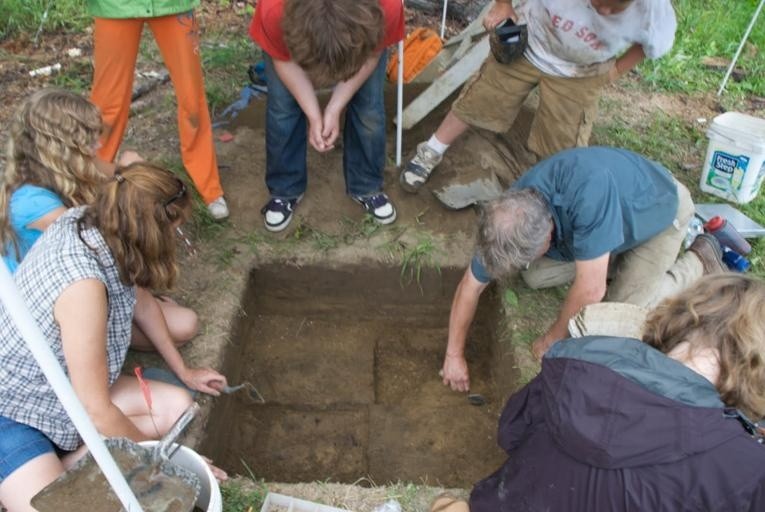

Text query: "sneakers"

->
[207,196,229,221]
[351,191,396,225]
[262,193,305,233]
[685,232,730,275]
[399,141,444,195]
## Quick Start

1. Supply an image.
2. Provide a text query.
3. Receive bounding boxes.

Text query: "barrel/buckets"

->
[567,302,651,340]
[700,111,765,204]
[131,441,223,512]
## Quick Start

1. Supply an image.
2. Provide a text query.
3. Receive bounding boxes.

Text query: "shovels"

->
[438,368,487,406]
[211,381,265,406]
[30,400,202,512]
